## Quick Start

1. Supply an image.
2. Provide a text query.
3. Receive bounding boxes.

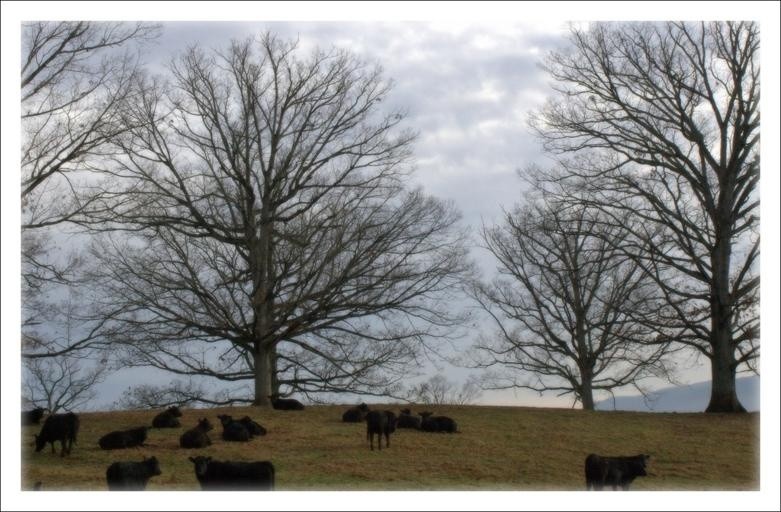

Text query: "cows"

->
[21,407,50,426]
[418,410,457,433]
[188,455,275,491]
[96,426,152,450]
[217,414,249,442]
[267,393,304,410]
[152,406,182,428]
[179,418,214,449]
[585,453,650,491]
[32,412,80,457]
[233,416,266,436]
[397,408,423,428]
[342,402,370,423]
[107,456,162,491]
[364,409,399,451]
